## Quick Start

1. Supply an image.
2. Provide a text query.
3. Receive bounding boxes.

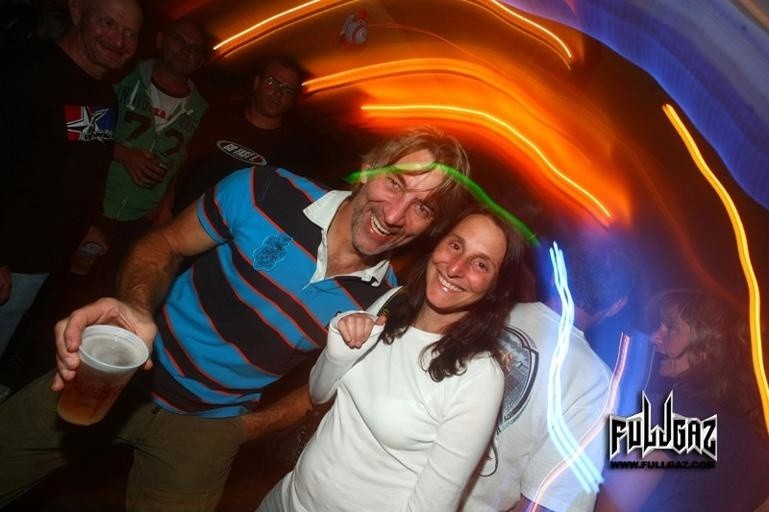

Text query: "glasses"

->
[259,67,299,100]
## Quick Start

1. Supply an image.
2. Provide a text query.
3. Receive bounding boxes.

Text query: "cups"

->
[56,324,149,426]
[71,241,104,275]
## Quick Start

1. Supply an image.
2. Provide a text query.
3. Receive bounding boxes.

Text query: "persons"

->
[454,220,644,511]
[0,0,143,362]
[588,286,760,511]
[0,17,209,406]
[1,125,474,511]
[153,51,312,232]
[253,203,528,511]
